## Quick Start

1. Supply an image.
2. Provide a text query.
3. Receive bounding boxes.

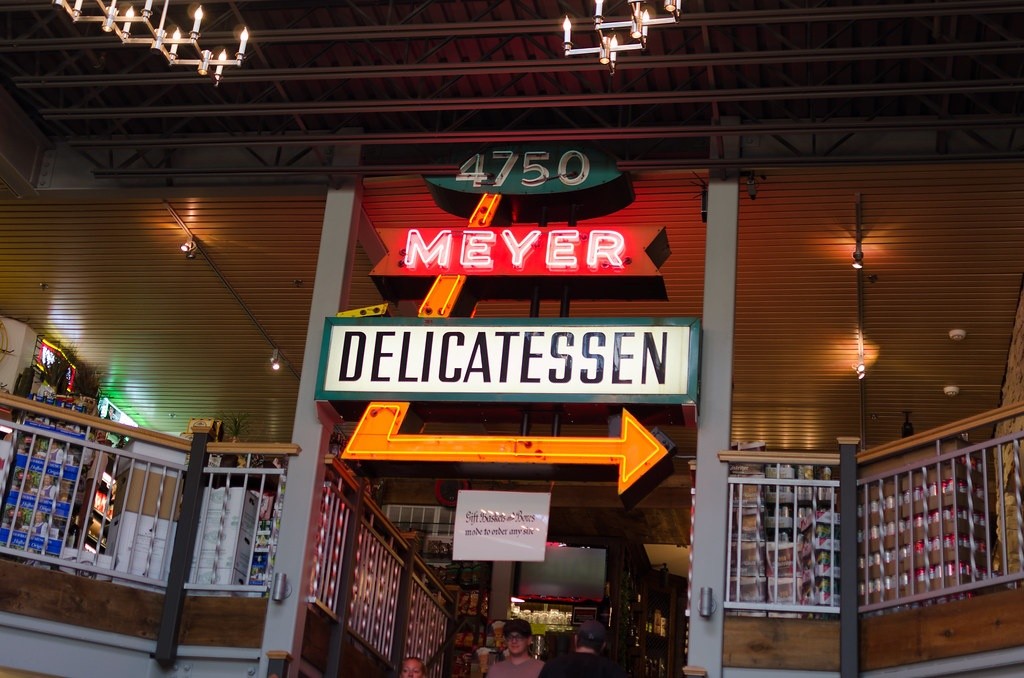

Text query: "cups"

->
[511,603,571,624]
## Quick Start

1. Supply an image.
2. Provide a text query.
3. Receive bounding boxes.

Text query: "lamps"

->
[562,0,685,74]
[180,240,199,258]
[850,246,866,269]
[271,350,280,370]
[850,363,866,380]
[54,0,248,83]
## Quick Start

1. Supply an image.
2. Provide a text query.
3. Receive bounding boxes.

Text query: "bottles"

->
[645,656,658,678]
[636,630,639,647]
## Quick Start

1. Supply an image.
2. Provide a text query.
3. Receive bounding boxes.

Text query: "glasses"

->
[505,633,526,641]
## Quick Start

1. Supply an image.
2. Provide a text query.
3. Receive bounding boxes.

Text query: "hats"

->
[503,619,532,639]
[576,620,606,643]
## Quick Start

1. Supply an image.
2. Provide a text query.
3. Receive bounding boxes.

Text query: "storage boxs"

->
[103,468,259,598]
[720,442,996,617]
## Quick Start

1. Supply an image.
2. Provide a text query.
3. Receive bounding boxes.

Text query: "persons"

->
[486,619,546,678]
[537,620,623,678]
[400,657,426,678]
